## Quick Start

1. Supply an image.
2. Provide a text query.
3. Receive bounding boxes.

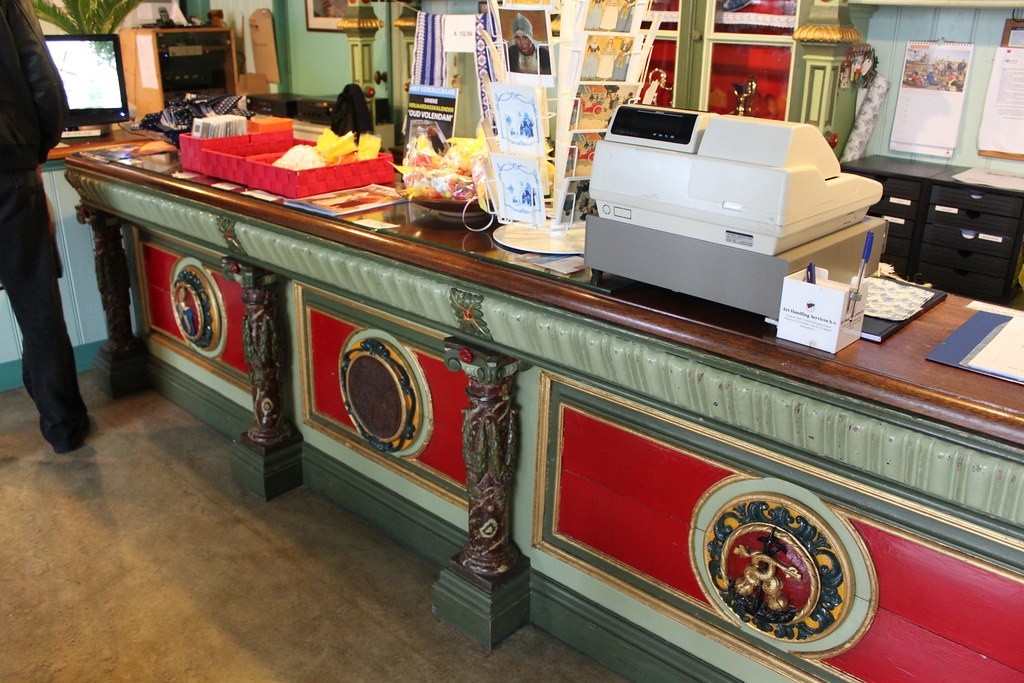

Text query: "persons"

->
[508,11,551,75]
[0,0,89,454]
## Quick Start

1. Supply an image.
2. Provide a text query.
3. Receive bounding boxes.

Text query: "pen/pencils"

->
[850,231,875,322]
[807,262,816,284]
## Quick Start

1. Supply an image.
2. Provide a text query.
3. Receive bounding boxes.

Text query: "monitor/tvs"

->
[44,34,130,131]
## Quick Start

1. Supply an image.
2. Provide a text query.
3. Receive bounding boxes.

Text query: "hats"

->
[508,12,541,49]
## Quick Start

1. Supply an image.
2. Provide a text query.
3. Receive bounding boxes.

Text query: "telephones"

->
[156,7,175,28]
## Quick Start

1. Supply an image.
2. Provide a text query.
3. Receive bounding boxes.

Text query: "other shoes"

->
[39,416,91,454]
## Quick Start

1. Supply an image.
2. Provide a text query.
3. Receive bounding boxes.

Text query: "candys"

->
[402,153,478,201]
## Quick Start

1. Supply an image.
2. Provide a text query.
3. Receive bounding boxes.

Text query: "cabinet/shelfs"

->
[385,0,878,161]
[119,27,238,119]
[841,153,1024,305]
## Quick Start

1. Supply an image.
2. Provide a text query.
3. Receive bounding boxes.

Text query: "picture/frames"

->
[304,0,346,33]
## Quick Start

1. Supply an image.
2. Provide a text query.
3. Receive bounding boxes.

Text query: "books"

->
[861,272,948,343]
